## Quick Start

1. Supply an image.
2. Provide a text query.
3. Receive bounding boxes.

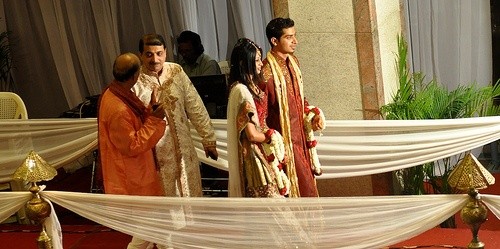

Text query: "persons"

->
[176,30,221,77]
[227,37,312,249]
[254,17,326,249]
[98,52,173,249]
[131,34,219,249]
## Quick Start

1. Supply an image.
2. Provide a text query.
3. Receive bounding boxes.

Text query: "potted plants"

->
[360,33,500,231]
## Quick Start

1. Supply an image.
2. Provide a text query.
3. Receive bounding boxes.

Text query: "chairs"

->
[0,92,38,224]
[217,61,232,87]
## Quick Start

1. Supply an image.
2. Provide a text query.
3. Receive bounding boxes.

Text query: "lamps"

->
[447,153,497,247]
[12,150,57,248]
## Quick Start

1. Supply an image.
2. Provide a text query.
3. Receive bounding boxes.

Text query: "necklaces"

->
[251,83,259,93]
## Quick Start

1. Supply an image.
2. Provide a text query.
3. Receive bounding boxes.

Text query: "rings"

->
[163,113,166,116]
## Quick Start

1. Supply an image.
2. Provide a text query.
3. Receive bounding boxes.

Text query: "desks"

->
[59,99,230,197]
